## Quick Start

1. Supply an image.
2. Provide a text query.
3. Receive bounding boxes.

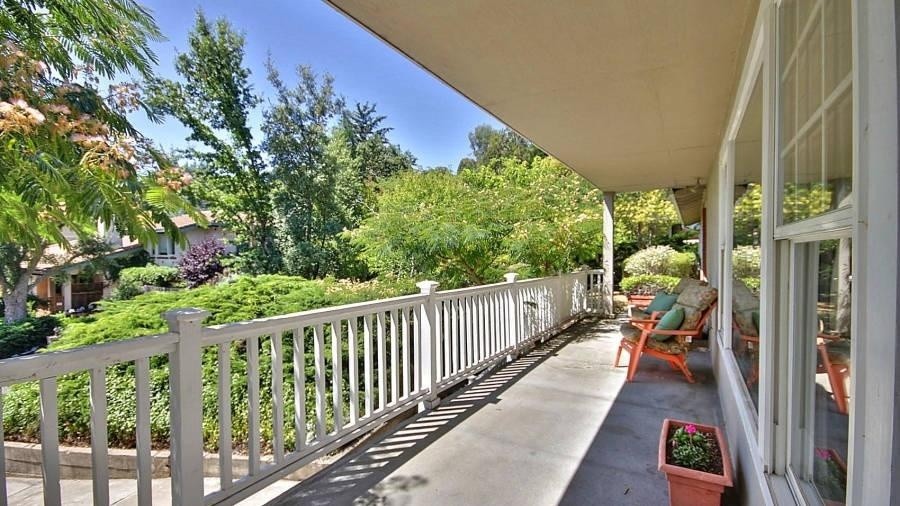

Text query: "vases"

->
[813,447,846,506]
[657,417,734,506]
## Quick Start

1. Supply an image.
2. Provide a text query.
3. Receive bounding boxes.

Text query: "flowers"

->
[669,423,712,472]
[815,449,839,488]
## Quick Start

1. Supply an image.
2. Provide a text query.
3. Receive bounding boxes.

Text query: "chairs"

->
[732,278,851,414]
[614,276,718,383]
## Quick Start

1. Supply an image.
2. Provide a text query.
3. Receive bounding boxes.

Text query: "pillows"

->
[752,312,759,335]
